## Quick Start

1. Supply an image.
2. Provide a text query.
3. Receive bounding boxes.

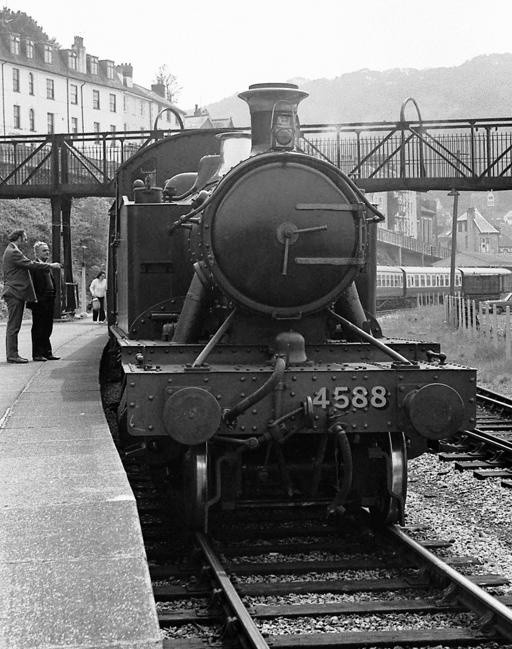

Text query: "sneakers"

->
[92,321,104,324]
[32,356,61,361]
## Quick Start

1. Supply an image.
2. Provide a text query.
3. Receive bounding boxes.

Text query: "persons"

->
[2,228,65,363]
[89,271,107,325]
[25,240,62,361]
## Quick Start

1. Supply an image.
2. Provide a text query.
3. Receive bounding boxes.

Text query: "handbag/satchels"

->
[87,301,92,310]
[92,297,101,310]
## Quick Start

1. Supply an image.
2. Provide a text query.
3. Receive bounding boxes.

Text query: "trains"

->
[107,83,511,534]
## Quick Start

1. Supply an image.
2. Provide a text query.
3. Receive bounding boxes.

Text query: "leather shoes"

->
[7,355,27,363]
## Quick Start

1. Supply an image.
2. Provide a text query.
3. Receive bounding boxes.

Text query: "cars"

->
[480,291,512,315]
